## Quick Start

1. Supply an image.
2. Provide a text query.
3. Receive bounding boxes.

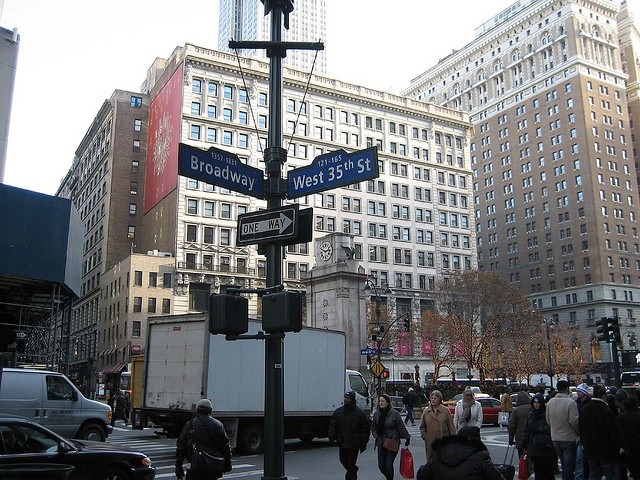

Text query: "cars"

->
[0,415,155,478]
[443,392,502,425]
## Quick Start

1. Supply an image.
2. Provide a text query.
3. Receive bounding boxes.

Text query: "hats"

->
[196,398,213,413]
[457,426,481,440]
[408,387,414,392]
[344,391,356,399]
[576,382,590,395]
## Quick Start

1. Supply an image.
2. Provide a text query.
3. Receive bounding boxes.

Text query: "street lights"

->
[546,318,555,386]
[364,278,392,327]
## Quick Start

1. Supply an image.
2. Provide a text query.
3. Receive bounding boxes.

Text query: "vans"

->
[0,367,112,441]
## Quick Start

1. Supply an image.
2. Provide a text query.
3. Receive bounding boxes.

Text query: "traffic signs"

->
[287,146,378,199]
[177,142,263,199]
[236,203,299,246]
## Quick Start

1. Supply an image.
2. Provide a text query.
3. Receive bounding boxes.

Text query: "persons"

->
[403,387,417,427]
[108,393,116,407]
[108,390,131,427]
[419,390,457,461]
[501,380,639,479]
[327,391,371,480]
[371,395,410,480]
[453,391,483,435]
[416,425,506,480]
[175,398,232,479]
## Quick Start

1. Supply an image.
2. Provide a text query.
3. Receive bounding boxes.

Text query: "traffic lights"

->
[403,317,410,332]
[208,292,248,335]
[594,316,608,341]
[382,370,389,378]
[261,290,302,333]
[607,315,619,339]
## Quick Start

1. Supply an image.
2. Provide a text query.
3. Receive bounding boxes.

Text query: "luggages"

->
[494,441,517,480]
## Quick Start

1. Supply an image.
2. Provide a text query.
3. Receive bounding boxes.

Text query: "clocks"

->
[318,242,333,262]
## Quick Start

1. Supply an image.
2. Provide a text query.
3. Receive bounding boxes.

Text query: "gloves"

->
[224,459,233,475]
[360,443,367,454]
[175,461,185,479]
[329,437,335,447]
[405,438,410,446]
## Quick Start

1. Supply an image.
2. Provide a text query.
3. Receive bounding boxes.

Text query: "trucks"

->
[143,313,374,454]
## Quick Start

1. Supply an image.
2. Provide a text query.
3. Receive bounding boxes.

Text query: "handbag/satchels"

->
[518,449,533,480]
[189,418,227,480]
[398,447,415,479]
[383,436,401,453]
[498,411,509,425]
